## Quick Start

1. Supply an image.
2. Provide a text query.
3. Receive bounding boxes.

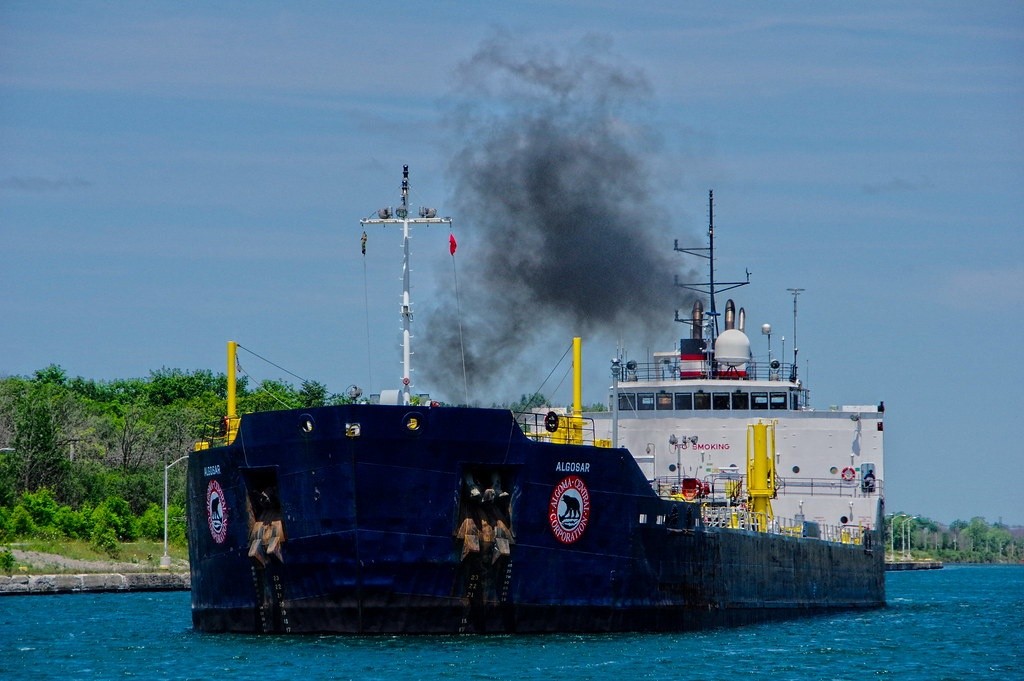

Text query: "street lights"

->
[907,516,919,561]
[901,516,917,561]
[891,514,906,562]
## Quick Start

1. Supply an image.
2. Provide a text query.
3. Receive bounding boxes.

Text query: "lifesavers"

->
[842,467,856,482]
[545,411,560,433]
[694,480,704,495]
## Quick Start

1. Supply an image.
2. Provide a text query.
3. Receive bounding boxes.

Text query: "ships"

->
[186,161,886,635]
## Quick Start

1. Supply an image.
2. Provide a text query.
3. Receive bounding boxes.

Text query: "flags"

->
[449,233,455,254]
[360,232,367,256]
